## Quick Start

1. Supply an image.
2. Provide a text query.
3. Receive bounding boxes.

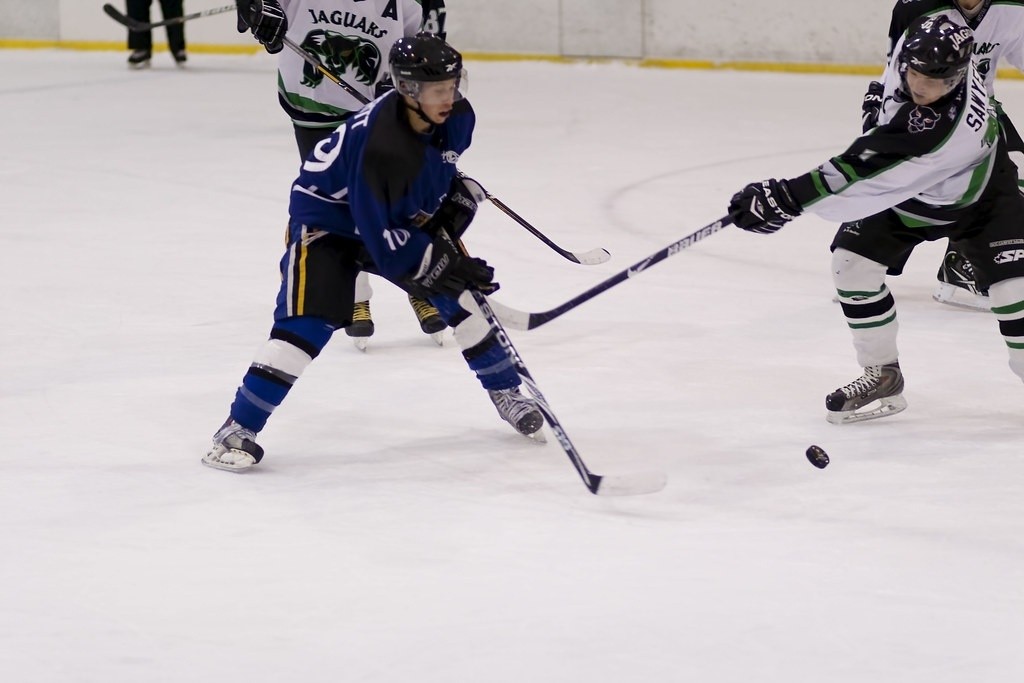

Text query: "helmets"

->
[390,32,463,81]
[899,15,974,80]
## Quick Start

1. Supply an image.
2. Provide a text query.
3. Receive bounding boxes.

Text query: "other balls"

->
[806,444,830,470]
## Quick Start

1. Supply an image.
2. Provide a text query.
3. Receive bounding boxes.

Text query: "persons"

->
[125,0,187,71]
[234,0,447,353]
[201,36,549,475]
[862,0,1024,311]
[728,14,1024,425]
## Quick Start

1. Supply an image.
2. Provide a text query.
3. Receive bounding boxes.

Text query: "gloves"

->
[408,246,497,293]
[862,81,885,131]
[424,178,478,239]
[728,177,802,233]
[235,0,292,54]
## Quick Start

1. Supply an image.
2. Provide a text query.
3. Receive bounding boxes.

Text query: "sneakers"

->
[344,301,374,349]
[173,51,187,67]
[486,383,548,444]
[127,49,151,68]
[407,291,449,346]
[826,361,908,423]
[201,419,266,470]
[933,242,993,310]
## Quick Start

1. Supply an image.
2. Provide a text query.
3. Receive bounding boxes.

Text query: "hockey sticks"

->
[278,33,611,266]
[435,225,668,496]
[457,210,746,333]
[102,2,238,33]
